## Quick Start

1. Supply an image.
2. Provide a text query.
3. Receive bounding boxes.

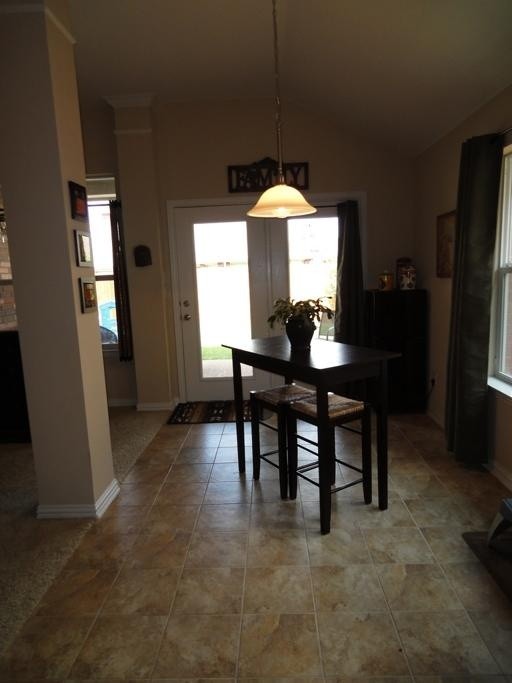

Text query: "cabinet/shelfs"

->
[395,256,410,287]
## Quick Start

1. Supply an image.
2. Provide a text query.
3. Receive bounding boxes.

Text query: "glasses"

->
[245,0,318,220]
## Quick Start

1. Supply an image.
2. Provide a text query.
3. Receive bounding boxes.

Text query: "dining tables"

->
[267,296,336,349]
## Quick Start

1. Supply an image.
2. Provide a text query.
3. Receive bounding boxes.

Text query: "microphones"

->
[288,391,374,505]
[249,382,317,499]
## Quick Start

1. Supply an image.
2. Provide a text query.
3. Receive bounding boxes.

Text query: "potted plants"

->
[68,181,89,222]
[74,229,93,268]
[79,276,98,314]
[435,210,458,278]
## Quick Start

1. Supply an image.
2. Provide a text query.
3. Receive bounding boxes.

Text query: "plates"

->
[365,289,429,414]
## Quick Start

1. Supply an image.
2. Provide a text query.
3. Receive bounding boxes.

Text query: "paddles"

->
[166,400,252,425]
